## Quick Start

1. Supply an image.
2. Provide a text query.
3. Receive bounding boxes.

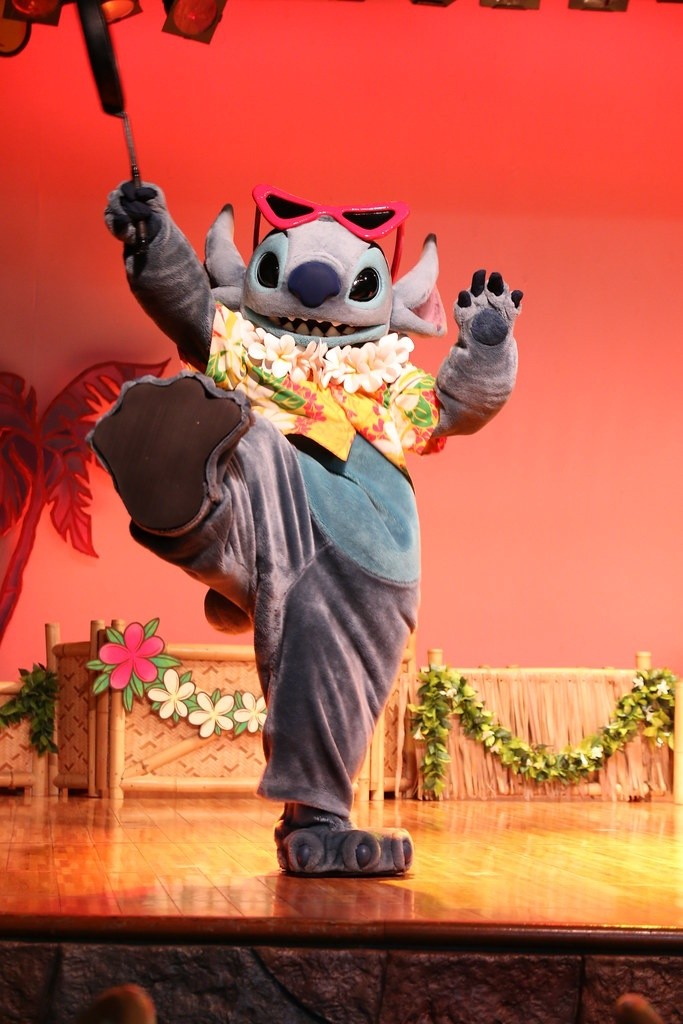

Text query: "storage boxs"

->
[0,677,47,796]
[382,649,683,804]
[45,618,385,800]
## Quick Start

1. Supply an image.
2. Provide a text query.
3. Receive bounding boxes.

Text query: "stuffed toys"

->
[84,180,524,877]
